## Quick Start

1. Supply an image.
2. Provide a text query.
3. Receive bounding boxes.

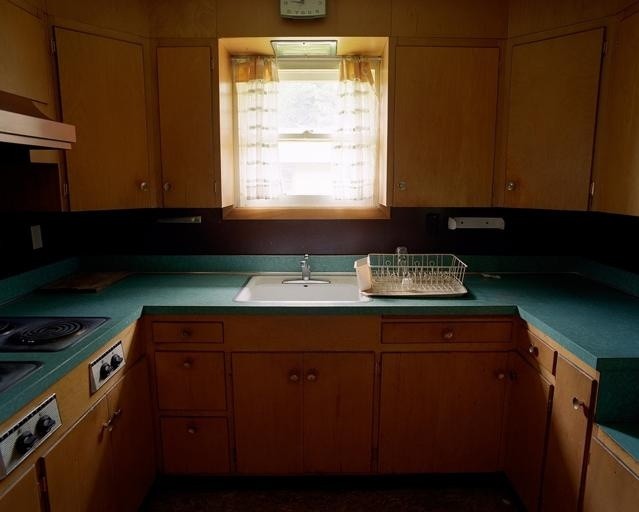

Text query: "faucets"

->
[300,254,310,281]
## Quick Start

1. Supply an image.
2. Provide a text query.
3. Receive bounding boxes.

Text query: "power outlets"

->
[426,212,440,237]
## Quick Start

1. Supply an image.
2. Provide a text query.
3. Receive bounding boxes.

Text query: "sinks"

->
[233,275,373,305]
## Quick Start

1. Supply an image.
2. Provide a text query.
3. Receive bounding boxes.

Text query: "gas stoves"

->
[0,315,127,458]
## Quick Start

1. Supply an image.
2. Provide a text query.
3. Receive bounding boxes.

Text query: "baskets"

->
[366,252,467,291]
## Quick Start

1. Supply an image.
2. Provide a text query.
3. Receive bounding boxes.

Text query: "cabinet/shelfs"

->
[0,0,53,102]
[0,463,42,511]
[0,17,164,213]
[505,17,614,212]
[280,0,327,20]
[538,352,598,512]
[579,434,637,512]
[149,316,231,478]
[391,38,506,207]
[227,349,376,475]
[589,1,638,217]
[148,39,235,209]
[375,316,513,475]
[39,353,154,510]
[507,324,558,511]
